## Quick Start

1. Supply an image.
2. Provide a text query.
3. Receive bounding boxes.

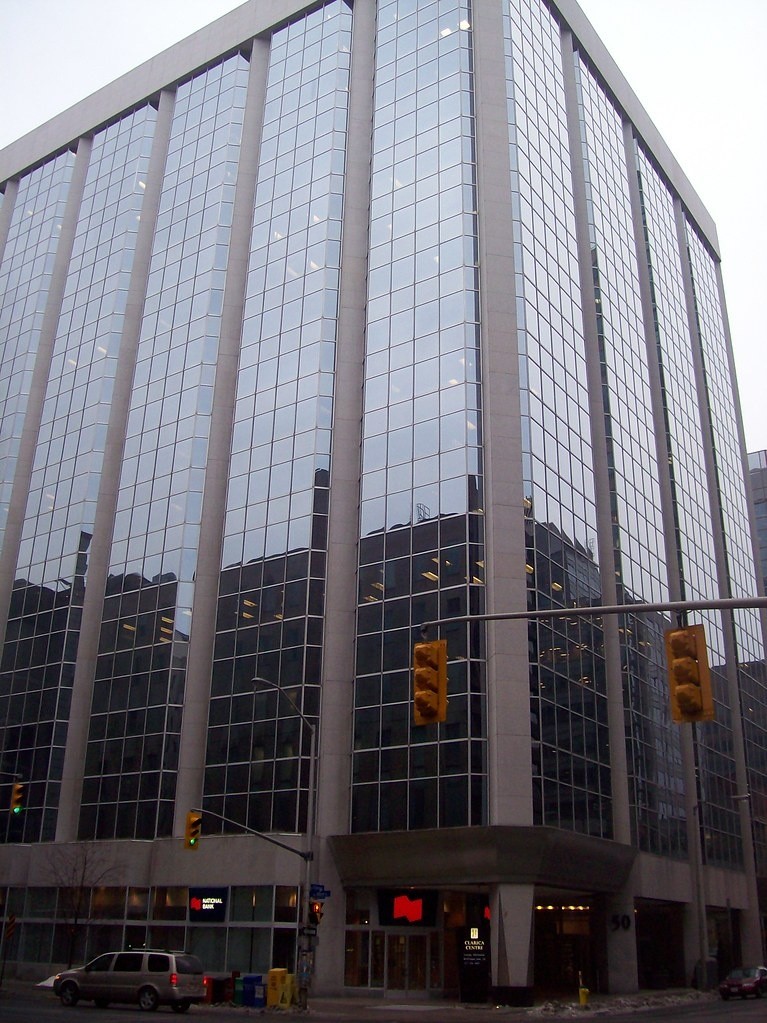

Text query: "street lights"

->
[252,676,315,1010]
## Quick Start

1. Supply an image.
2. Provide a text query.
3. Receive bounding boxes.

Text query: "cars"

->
[719,966,767,1000]
[53,949,207,1013]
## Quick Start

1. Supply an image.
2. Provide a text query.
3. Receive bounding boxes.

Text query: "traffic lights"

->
[183,813,202,850]
[10,783,25,814]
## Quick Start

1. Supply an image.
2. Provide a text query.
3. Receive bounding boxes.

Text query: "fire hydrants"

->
[578,985,591,1010]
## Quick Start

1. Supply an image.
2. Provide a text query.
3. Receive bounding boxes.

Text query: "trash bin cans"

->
[205,967,288,1007]
